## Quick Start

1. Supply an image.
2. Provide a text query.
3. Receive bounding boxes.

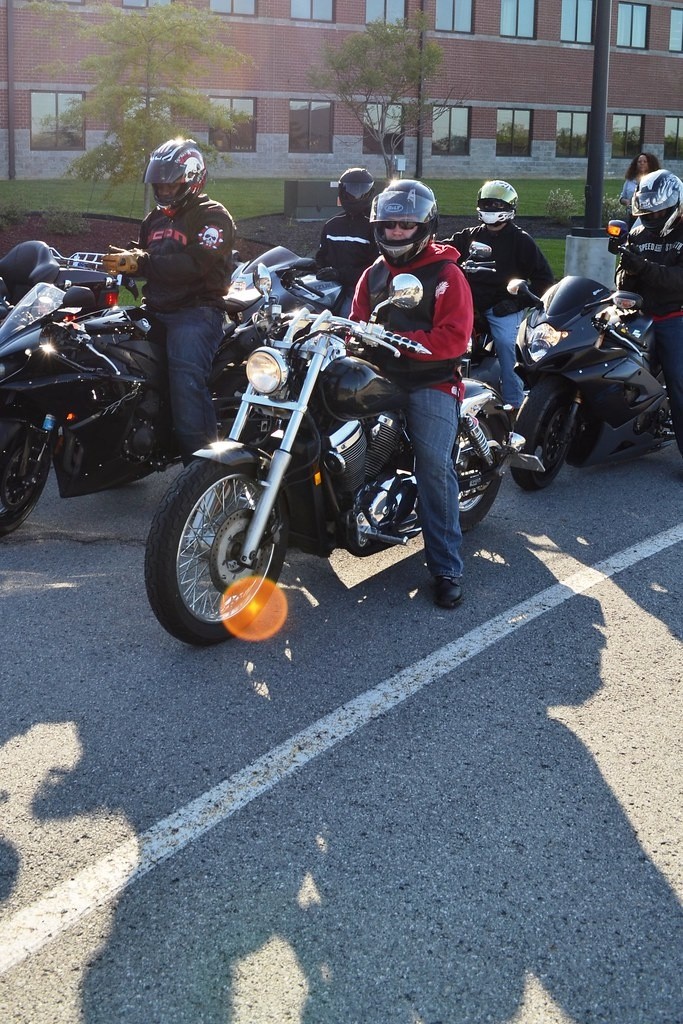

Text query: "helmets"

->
[337,168,375,219]
[476,179,518,224]
[369,179,438,268]
[631,169,683,234]
[142,139,207,216]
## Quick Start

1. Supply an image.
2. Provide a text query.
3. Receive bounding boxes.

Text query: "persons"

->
[101,138,238,469]
[342,177,474,608]
[619,152,660,222]
[316,168,381,319]
[436,180,555,410]
[613,169,683,459]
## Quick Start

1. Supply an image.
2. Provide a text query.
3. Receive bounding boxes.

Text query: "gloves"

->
[492,300,519,316]
[128,247,146,255]
[617,243,645,276]
[316,266,339,281]
[102,245,138,274]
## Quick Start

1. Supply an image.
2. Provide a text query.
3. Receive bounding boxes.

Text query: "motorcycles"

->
[456,243,519,390]
[505,275,677,494]
[0,239,347,538]
[143,262,544,648]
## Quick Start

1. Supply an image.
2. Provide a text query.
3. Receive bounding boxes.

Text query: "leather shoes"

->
[434,575,462,607]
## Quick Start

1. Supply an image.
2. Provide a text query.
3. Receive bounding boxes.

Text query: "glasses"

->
[382,220,419,230]
